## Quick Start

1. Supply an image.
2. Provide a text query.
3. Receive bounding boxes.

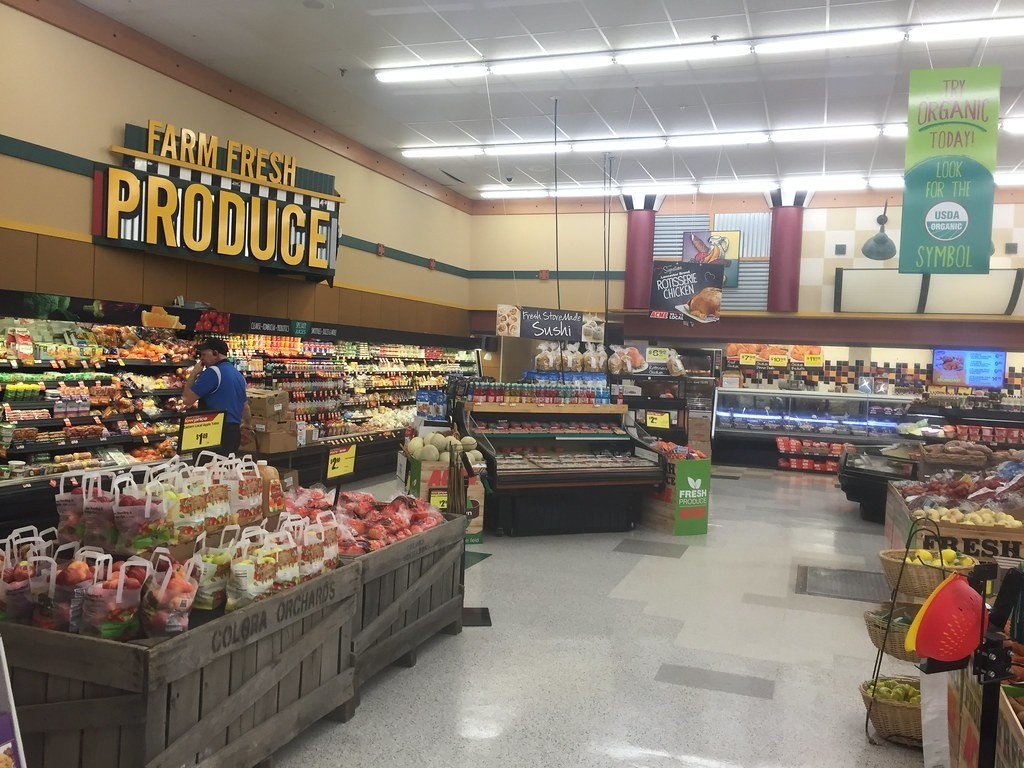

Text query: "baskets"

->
[859,673,922,742]
[864,604,923,663]
[878,548,979,596]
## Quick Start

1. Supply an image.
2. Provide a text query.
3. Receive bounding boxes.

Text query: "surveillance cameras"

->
[506,178,512,181]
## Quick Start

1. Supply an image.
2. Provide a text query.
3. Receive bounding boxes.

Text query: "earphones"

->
[213,352,217,356]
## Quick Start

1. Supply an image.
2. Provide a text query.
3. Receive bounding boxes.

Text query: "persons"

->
[182,338,253,467]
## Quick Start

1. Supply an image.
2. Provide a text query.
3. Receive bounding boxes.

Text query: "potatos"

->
[899,440,1024,505]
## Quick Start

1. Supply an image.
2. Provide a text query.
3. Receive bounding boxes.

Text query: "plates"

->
[675,305,719,324]
[633,362,648,373]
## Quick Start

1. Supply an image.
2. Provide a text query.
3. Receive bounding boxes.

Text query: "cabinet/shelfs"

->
[453,400,669,536]
[0,359,483,522]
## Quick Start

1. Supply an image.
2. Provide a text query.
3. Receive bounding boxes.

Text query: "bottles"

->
[468,381,611,404]
[193,332,349,435]
[617,387,623,404]
[926,389,1024,413]
[251,460,283,517]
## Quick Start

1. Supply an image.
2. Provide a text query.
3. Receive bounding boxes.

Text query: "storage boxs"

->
[275,464,298,492]
[1,503,470,768]
[637,457,711,535]
[235,388,298,454]
[885,480,1024,559]
[947,652,1024,768]
[397,441,487,544]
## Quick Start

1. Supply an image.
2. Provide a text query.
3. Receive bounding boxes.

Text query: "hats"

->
[194,338,228,356]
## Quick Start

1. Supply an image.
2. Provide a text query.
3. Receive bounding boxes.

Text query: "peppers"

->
[866,678,922,705]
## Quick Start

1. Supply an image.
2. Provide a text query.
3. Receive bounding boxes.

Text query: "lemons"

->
[905,547,975,568]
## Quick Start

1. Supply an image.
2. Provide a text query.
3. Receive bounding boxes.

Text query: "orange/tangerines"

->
[141,306,186,329]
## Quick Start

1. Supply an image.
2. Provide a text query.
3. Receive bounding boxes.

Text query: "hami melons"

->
[407,432,484,465]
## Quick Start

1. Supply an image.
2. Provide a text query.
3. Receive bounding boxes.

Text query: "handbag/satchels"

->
[0,451,338,643]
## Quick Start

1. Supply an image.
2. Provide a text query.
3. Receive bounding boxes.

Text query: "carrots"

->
[995,637,1024,733]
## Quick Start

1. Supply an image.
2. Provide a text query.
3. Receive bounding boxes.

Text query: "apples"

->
[89,326,198,360]
[616,346,644,367]
[912,507,1023,529]
[130,433,178,463]
[0,483,334,643]
[195,311,230,333]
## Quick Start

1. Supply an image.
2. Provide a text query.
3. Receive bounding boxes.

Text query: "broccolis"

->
[21,292,70,319]
